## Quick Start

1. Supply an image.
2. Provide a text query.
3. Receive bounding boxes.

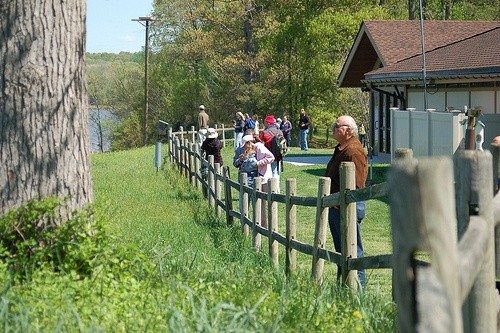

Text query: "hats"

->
[240,135,253,148]
[205,128,218,138]
[199,105,204,110]
[264,116,275,124]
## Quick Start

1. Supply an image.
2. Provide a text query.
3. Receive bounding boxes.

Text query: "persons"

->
[198,105,311,228]
[325,115,368,294]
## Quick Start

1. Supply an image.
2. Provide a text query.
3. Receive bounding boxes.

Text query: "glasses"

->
[334,123,349,128]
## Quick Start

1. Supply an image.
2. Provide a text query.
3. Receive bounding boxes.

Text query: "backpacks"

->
[248,119,255,129]
[266,129,287,156]
